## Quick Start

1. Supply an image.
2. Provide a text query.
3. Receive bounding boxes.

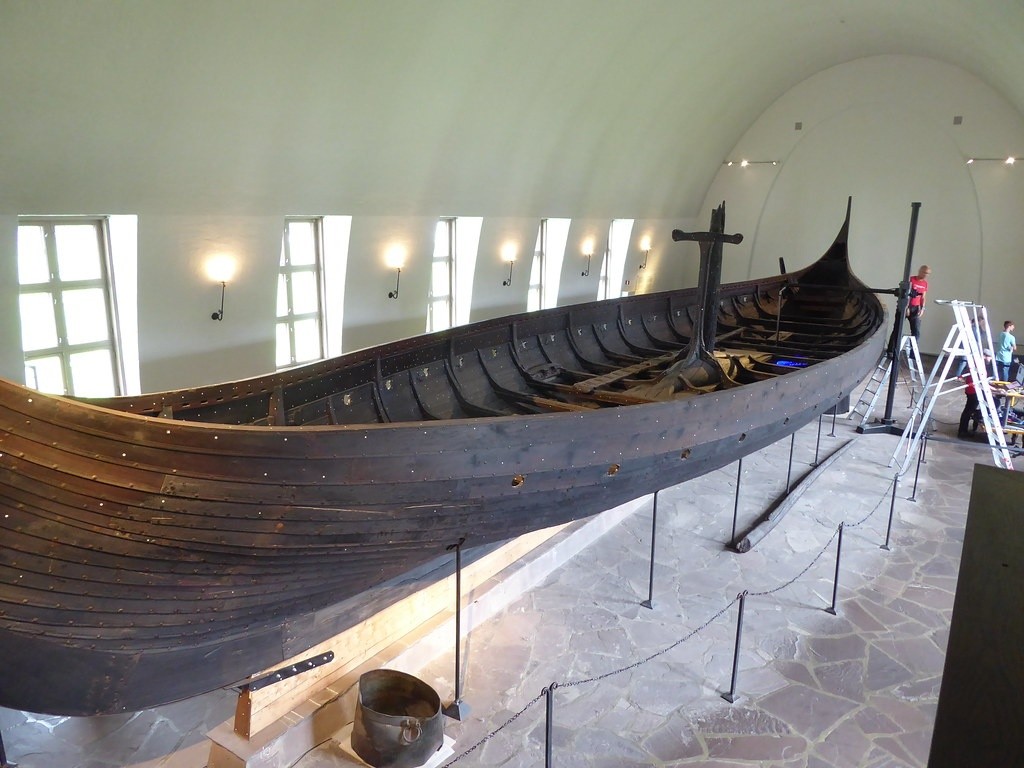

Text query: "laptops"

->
[1004,363,1024,389]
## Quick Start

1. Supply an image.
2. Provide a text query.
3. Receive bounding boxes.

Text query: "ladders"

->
[847,336,941,432]
[888,297,1014,480]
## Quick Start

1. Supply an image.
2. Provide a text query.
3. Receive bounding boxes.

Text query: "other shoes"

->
[958,431,974,438]
[955,378,965,382]
[887,351,894,359]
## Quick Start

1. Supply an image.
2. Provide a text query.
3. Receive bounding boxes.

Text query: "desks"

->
[978,381,1024,446]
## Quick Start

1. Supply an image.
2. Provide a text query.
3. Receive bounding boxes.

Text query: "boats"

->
[1,196,893,716]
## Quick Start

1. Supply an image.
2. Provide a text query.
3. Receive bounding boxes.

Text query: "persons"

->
[956,315,986,382]
[887,265,931,360]
[958,349,992,438]
[992,321,1017,382]
